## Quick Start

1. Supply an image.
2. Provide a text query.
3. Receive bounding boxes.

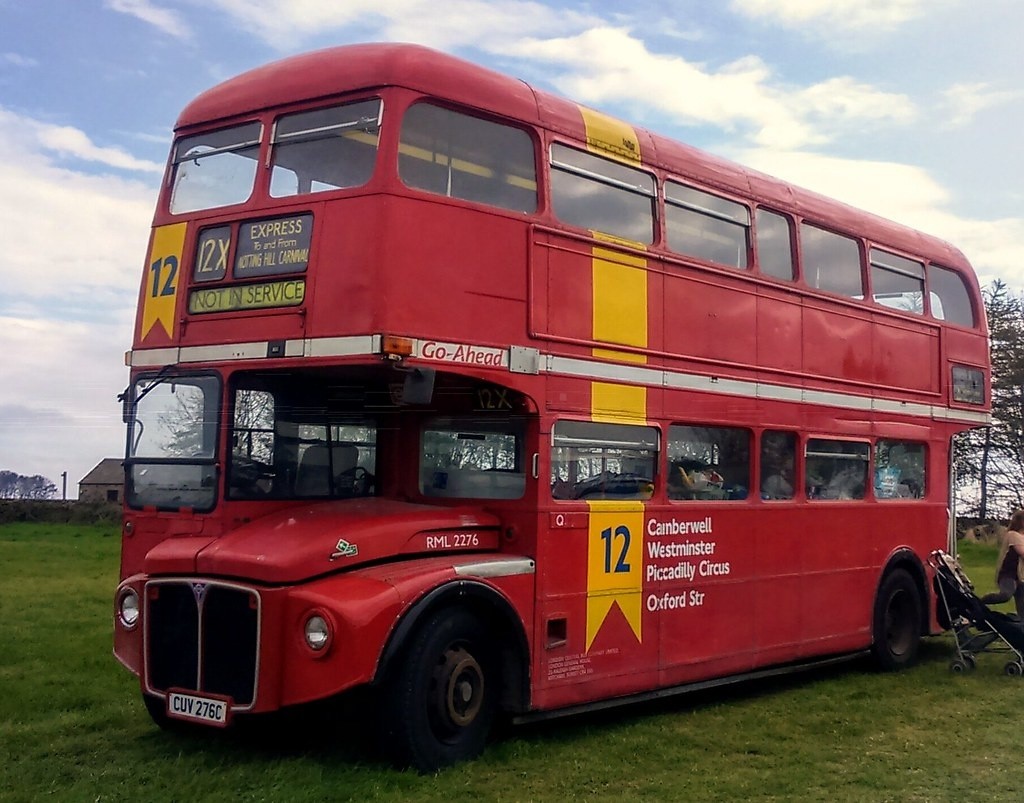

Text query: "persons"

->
[981,510,1024,619]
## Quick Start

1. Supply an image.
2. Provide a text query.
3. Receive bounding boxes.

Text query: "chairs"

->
[296,443,358,497]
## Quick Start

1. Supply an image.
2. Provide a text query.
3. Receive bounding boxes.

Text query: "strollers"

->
[927,548,1024,677]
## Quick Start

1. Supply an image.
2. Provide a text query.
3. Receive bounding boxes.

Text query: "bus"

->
[109,42,991,777]
